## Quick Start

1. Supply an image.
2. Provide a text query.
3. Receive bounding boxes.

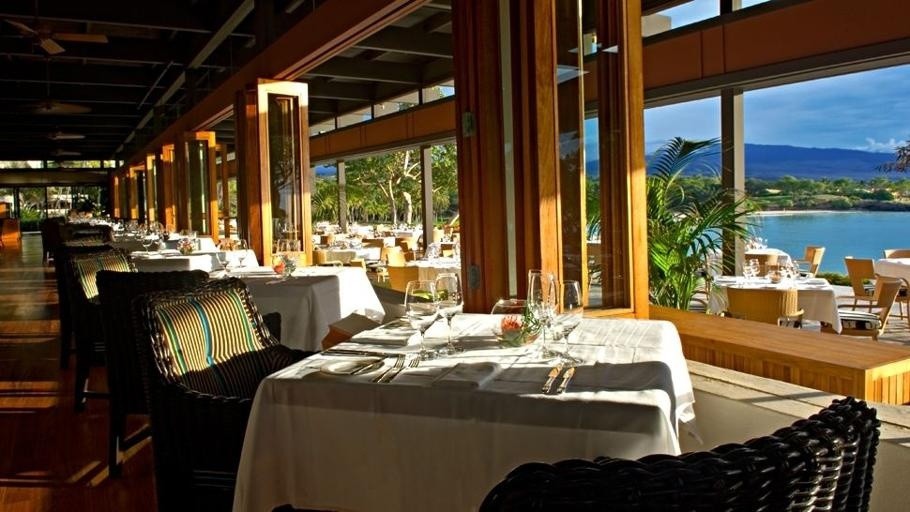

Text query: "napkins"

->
[432,362,501,389]
[350,329,421,346]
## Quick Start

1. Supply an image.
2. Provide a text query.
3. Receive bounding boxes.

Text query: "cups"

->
[426,244,442,258]
[123,218,200,258]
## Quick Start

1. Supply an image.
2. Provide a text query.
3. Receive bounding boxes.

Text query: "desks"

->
[230,312,698,511]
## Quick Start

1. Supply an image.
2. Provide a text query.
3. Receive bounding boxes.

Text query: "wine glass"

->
[276,237,302,282]
[434,273,465,355]
[742,236,800,284]
[215,239,248,273]
[403,279,441,361]
[528,268,584,346]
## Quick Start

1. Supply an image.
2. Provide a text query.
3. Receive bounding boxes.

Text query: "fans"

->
[0,0,109,58]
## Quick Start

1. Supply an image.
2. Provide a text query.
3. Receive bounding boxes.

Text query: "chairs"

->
[37,213,464,412]
[133,275,324,511]
[698,237,909,342]
[95,270,208,479]
[478,397,881,511]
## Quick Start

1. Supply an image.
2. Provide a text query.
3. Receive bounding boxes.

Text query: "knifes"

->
[541,362,564,393]
[556,367,575,393]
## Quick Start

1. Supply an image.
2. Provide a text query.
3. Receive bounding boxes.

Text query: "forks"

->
[388,359,419,383]
[372,354,405,383]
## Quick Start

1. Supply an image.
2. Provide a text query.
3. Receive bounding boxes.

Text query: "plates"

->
[320,356,384,376]
